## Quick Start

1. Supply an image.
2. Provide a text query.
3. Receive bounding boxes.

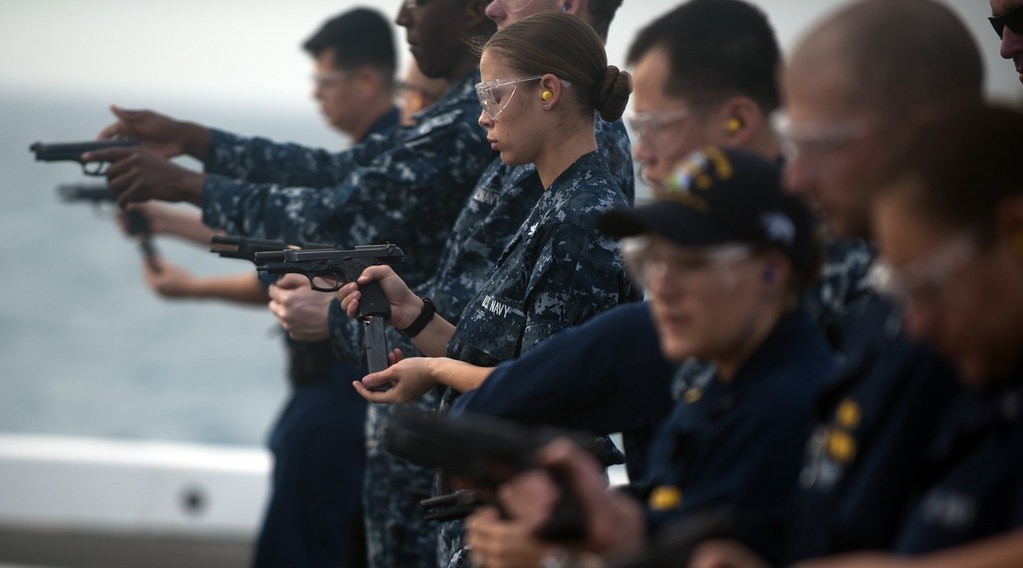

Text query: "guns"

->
[382,402,606,543]
[30,135,147,201]
[209,233,286,265]
[254,240,409,392]
[56,184,161,272]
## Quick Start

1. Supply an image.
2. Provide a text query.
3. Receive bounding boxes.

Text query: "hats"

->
[592,145,816,277]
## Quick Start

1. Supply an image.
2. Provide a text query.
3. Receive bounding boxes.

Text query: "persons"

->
[79,0,1023,568]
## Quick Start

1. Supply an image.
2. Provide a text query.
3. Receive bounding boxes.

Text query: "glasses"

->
[621,238,755,285]
[473,73,572,120]
[622,89,762,142]
[987,3,1023,40]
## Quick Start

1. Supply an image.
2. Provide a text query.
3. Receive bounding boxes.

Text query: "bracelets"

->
[395,297,437,338]
